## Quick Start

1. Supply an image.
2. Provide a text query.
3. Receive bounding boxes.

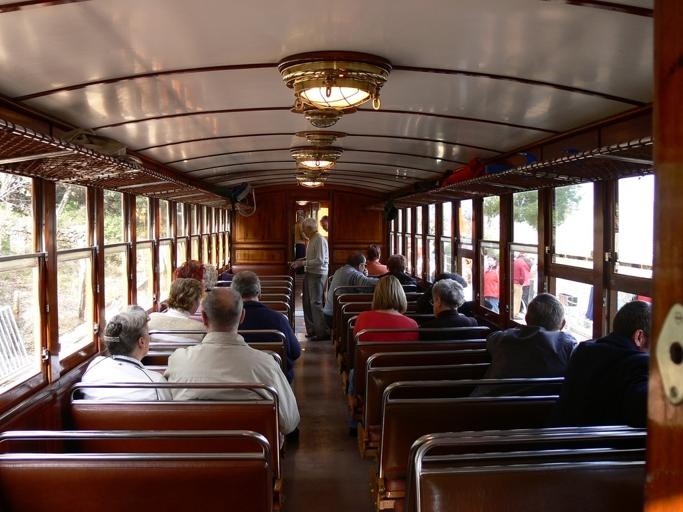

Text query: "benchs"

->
[137,329,289,377]
[215,275,294,283]
[331,286,415,344]
[253,286,293,301]
[70,383,281,507]
[373,379,563,509]
[348,328,486,421]
[405,424,651,512]
[329,273,400,281]
[340,313,468,400]
[256,300,291,319]
[207,280,293,287]
[358,351,492,457]
[333,292,428,358]
[0,426,275,512]
[337,302,450,377]
[254,294,292,302]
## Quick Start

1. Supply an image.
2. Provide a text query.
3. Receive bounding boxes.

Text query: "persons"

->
[417,273,467,315]
[171,259,207,315]
[346,275,418,410]
[288,217,331,341]
[378,254,415,285]
[419,278,481,340]
[477,248,542,316]
[147,277,207,345]
[549,300,650,428]
[469,292,577,397]
[319,216,329,232]
[322,253,379,328]
[165,287,301,450]
[294,216,309,274]
[79,305,171,402]
[229,271,301,384]
[364,244,388,276]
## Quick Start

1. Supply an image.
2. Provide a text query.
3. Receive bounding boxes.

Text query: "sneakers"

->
[312,334,331,342]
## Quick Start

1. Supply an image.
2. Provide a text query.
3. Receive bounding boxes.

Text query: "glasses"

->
[360,263,368,268]
[429,298,438,306]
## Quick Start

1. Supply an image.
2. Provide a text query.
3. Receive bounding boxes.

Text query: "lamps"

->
[276,47,392,125]
[287,131,352,171]
[298,174,334,189]
